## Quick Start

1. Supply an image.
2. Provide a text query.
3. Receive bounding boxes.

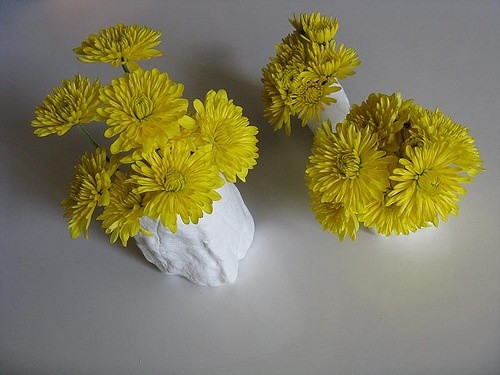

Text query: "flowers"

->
[306,96,480,239]
[258,11,361,137]
[31,23,259,246]
